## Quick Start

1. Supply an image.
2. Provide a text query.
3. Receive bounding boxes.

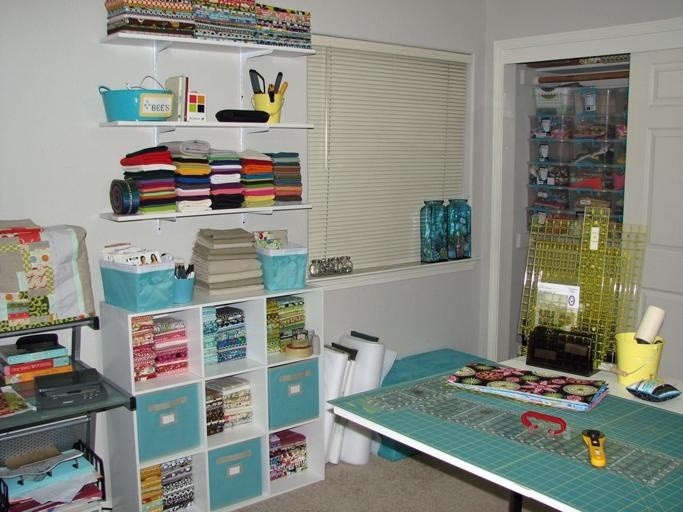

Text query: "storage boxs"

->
[137,382,198,462]
[209,437,259,511]
[526,86,628,232]
[255,241,307,290]
[100,259,194,312]
[269,358,318,432]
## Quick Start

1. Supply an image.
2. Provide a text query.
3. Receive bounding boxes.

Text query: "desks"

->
[333,349,683,512]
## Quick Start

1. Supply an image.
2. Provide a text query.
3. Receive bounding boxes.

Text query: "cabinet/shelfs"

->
[1,320,136,509]
[99,30,313,224]
[96,285,324,510]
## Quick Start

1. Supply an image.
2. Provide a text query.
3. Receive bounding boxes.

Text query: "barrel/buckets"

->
[251,93,285,124]
[609,332,665,388]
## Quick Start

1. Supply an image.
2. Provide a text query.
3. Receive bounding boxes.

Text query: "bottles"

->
[418,196,471,264]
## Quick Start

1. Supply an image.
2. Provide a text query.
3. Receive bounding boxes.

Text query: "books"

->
[165,75,189,121]
[0,344,73,386]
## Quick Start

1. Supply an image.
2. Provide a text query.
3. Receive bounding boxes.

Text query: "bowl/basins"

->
[100,89,173,121]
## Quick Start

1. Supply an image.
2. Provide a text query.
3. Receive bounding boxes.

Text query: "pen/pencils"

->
[247,67,288,102]
